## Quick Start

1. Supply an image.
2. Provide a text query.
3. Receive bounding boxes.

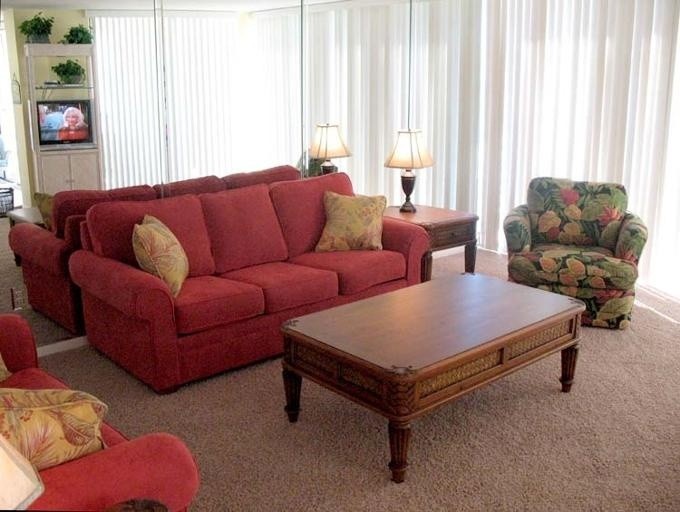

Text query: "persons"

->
[57,107,88,140]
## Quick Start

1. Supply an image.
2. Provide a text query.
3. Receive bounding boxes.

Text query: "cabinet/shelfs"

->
[23,43,103,195]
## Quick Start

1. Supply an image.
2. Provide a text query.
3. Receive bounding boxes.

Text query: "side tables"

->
[382,203,479,283]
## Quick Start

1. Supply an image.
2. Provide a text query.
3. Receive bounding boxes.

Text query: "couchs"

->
[9,215,87,335]
[0,312,199,512]
[68,217,431,395]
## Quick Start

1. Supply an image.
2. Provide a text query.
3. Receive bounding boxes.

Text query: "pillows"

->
[0,354,12,382]
[33,165,298,237]
[0,389,108,470]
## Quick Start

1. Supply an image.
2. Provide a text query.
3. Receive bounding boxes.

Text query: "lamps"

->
[308,123,352,174]
[384,128,435,213]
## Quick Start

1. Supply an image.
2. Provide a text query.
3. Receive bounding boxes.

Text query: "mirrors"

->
[0,0,481,358]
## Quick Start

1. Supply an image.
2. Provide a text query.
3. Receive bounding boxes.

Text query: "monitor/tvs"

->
[32,97,98,151]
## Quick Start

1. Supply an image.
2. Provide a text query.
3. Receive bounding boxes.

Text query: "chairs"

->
[504,176,647,331]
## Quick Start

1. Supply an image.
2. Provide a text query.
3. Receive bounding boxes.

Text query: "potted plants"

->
[10,12,114,107]
[17,11,55,44]
[52,58,86,84]
